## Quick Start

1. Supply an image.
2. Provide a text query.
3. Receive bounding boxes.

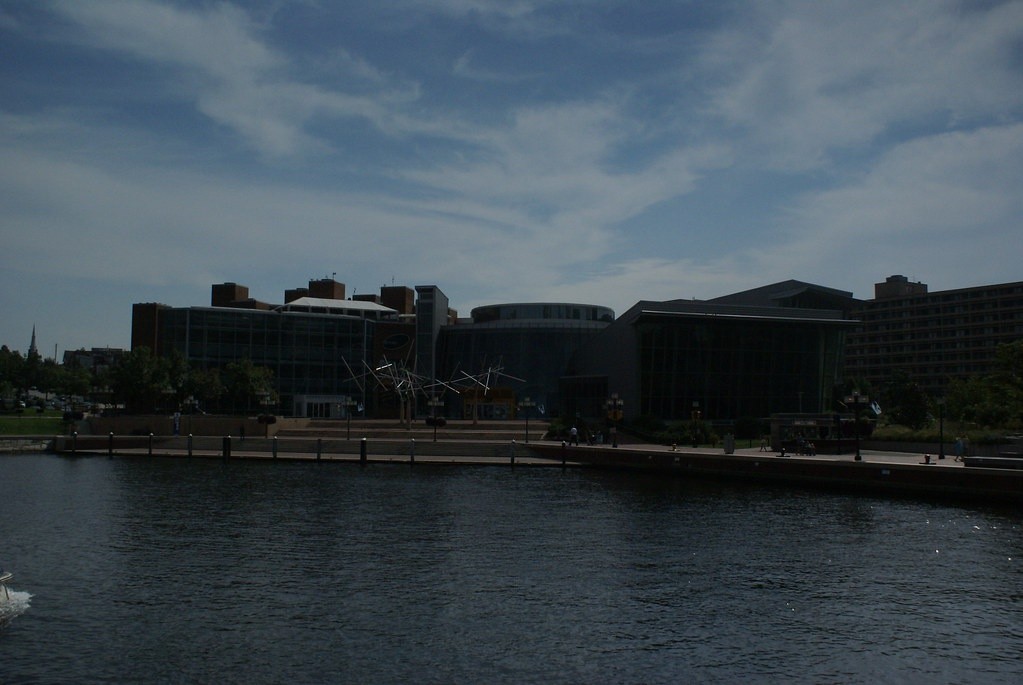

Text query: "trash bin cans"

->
[723,433,735,454]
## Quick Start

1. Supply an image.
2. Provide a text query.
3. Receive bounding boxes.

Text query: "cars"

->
[19,401,26,408]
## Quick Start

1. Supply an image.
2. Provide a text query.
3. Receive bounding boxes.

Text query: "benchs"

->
[800,448,827,455]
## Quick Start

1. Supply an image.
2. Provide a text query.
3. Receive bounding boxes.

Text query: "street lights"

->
[937,396,945,459]
[428,396,444,441]
[518,397,536,443]
[607,393,623,448]
[342,396,357,440]
[184,395,198,434]
[796,392,805,413]
[161,385,177,415]
[260,395,275,438]
[853,391,861,460]
[692,400,699,448]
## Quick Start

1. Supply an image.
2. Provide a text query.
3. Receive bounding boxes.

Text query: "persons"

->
[569,425,578,446]
[797,432,816,456]
[240,424,245,440]
[587,428,603,446]
[954,432,970,462]
[759,433,767,452]
